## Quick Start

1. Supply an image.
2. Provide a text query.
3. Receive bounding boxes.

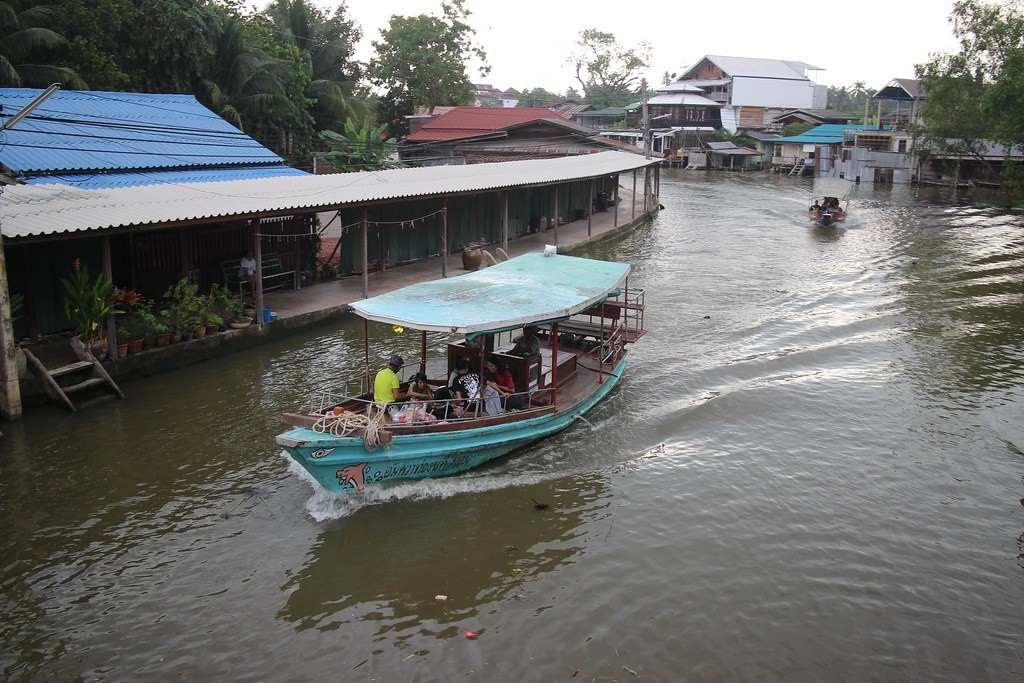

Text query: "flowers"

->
[112,288,124,305]
[125,292,139,307]
[75,259,81,270]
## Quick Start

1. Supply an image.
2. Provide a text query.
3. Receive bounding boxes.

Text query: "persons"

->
[374,356,414,413]
[408,371,435,409]
[451,360,509,413]
[239,249,259,299]
[482,355,522,409]
[507,324,542,357]
[427,390,461,423]
[809,197,842,220]
[445,358,463,388]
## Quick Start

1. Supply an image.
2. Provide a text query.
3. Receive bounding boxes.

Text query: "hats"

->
[390,355,404,366]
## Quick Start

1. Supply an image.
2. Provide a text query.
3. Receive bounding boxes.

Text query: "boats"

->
[272,241,646,496]
[807,177,853,227]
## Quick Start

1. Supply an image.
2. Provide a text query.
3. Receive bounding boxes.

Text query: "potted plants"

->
[131,276,252,350]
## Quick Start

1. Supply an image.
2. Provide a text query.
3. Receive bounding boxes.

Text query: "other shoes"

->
[251,291,256,299]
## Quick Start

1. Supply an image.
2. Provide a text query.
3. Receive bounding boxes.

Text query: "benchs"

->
[537,304,624,363]
[220,253,296,300]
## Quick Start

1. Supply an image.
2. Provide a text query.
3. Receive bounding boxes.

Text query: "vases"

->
[71,332,107,362]
[117,344,127,358]
[129,338,144,354]
